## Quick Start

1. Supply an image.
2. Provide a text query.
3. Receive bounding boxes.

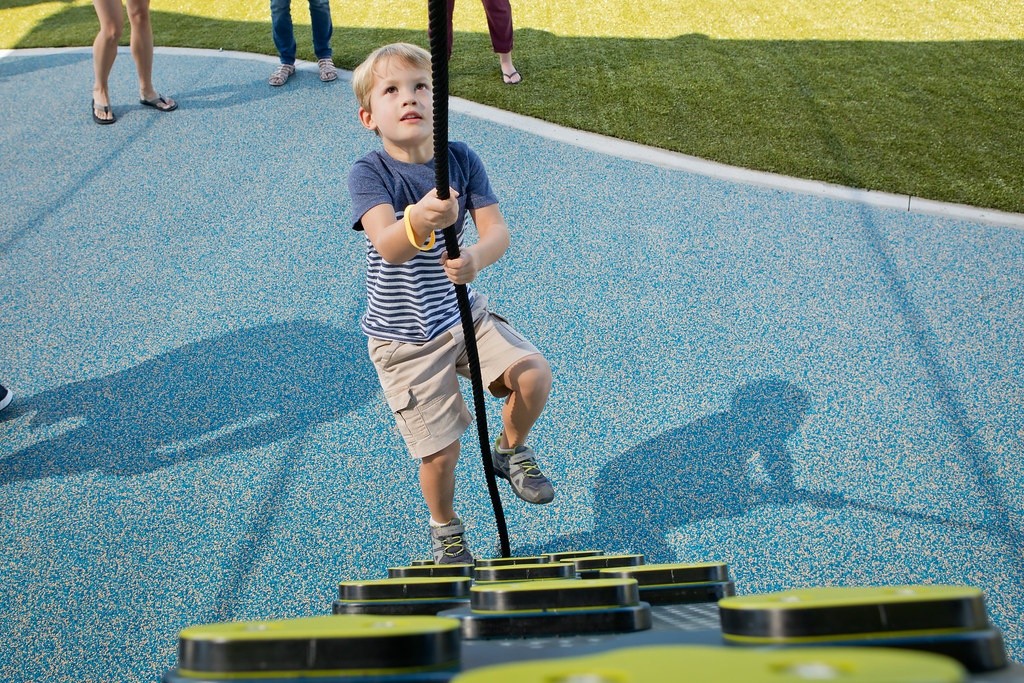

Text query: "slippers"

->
[91,98,117,124]
[140,94,178,111]
[502,71,523,84]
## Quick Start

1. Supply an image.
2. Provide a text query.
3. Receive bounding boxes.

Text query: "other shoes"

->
[0,384,13,411]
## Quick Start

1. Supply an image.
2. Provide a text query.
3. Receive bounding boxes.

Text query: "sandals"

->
[269,64,295,86]
[318,59,338,82]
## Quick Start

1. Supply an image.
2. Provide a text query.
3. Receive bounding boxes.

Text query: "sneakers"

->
[429,512,473,565]
[491,432,554,504]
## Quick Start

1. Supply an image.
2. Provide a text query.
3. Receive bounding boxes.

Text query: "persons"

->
[341,42,554,569]
[428,0,524,86]
[268,0,338,86]
[91,0,179,124]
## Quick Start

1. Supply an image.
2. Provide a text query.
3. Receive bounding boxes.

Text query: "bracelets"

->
[404,203,436,252]
